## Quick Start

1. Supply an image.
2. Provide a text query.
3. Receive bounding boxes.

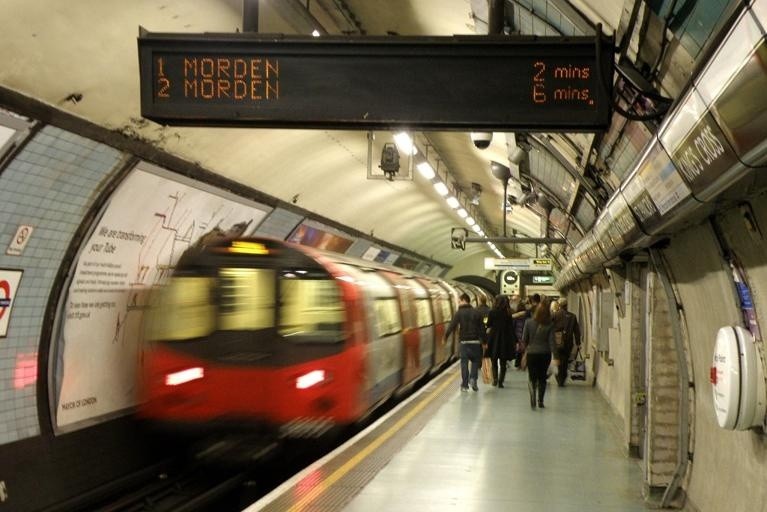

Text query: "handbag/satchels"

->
[481,358,493,384]
[554,331,565,346]
[568,348,586,381]
[520,350,527,370]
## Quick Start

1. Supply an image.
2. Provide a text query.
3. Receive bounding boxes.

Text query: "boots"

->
[538,383,546,408]
[528,382,536,408]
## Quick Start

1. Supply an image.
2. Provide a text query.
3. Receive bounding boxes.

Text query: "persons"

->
[475,289,583,389]
[439,292,489,393]
[518,303,561,411]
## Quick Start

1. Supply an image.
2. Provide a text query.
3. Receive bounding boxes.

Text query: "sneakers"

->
[470,378,478,391]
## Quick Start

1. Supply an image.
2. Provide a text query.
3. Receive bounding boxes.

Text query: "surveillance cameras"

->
[471,132,493,149]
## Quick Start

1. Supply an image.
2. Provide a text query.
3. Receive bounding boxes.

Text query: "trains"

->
[132,233,498,493]
